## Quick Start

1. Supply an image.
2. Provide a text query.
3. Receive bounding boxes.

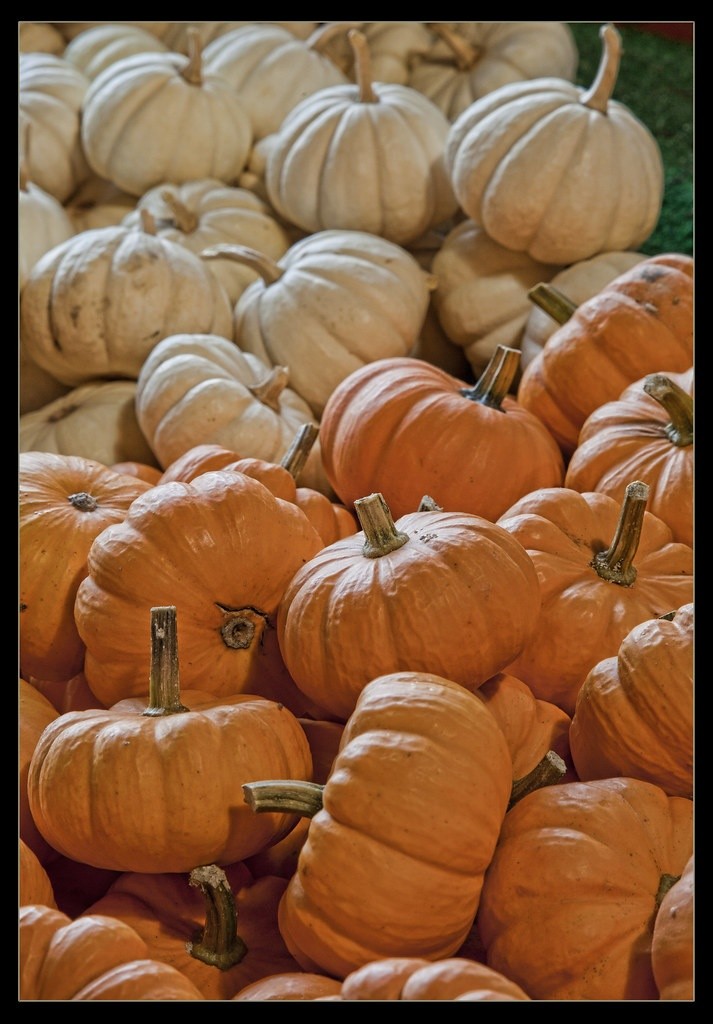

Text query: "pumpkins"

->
[17,23,694,1000]
